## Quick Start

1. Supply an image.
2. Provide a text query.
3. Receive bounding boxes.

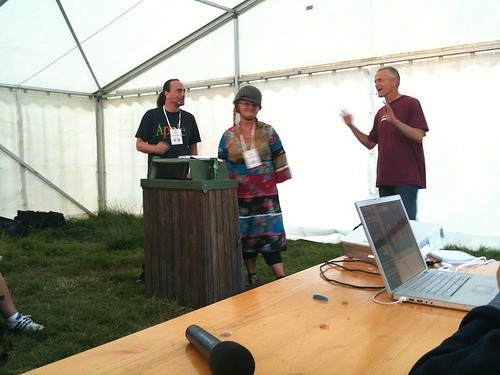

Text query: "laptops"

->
[355,195,500,312]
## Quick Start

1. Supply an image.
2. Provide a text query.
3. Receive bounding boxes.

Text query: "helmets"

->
[233,85,262,110]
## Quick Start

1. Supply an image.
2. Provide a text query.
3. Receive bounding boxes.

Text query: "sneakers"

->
[137,270,144,283]
[249,272,261,284]
[7,314,46,332]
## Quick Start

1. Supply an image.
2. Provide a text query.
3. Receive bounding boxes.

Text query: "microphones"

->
[186,325,255,375]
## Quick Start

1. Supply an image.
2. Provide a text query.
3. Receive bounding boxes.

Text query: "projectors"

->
[342,219,446,266]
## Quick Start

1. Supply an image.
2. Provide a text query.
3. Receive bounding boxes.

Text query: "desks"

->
[23,256,500,375]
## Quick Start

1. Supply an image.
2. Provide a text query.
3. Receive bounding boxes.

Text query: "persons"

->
[340,66,429,220]
[218,86,291,287]
[135,79,201,285]
[0,273,44,332]
